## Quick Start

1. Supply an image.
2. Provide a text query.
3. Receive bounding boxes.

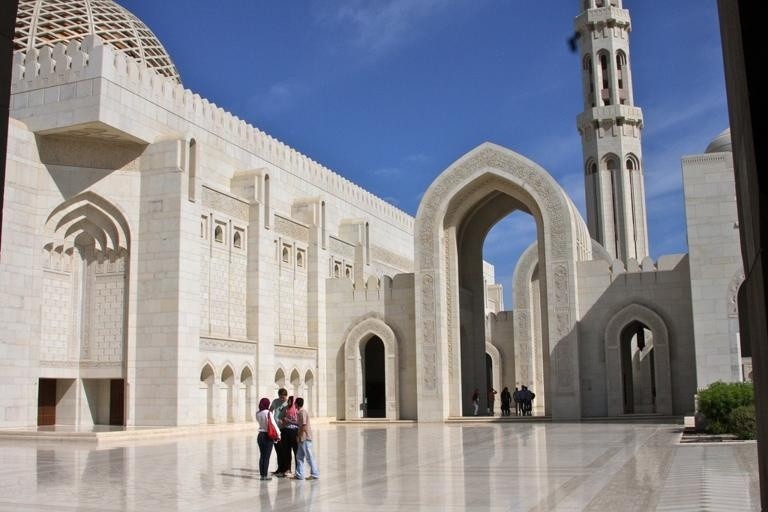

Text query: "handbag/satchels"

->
[267,411,277,440]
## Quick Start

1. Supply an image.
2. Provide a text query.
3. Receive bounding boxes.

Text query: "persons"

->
[255,397,275,480]
[292,397,320,481]
[470,383,535,416]
[269,388,288,474]
[281,396,296,474]
